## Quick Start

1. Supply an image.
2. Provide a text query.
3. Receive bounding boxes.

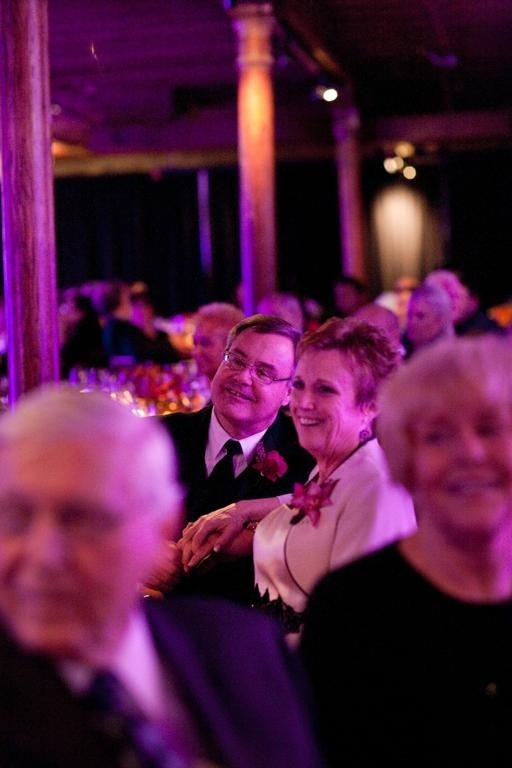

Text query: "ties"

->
[85,669,188,766]
[208,441,243,497]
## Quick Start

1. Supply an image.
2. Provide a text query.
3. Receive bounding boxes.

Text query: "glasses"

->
[222,347,291,385]
